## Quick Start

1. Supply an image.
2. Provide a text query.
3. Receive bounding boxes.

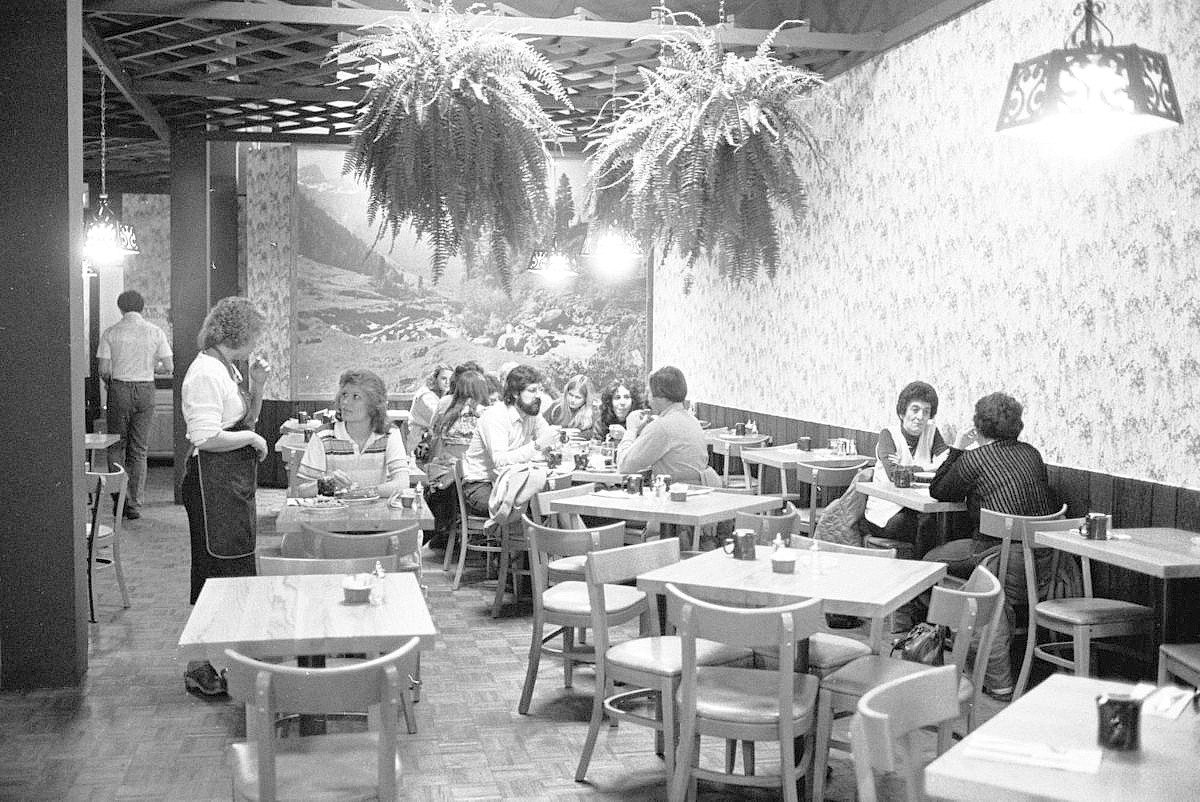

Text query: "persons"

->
[592,381,642,442]
[864,381,950,627]
[402,363,596,595]
[180,296,272,695]
[295,369,410,497]
[911,392,1054,626]
[616,366,709,485]
[97,290,174,518]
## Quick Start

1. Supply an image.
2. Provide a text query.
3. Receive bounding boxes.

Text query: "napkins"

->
[288,498,313,505]
[1071,528,1131,540]
[968,734,1103,774]
[1133,681,1195,721]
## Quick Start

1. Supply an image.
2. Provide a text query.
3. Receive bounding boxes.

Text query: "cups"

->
[798,436,812,452]
[1095,693,1141,752]
[574,454,589,470]
[895,470,911,488]
[735,423,745,437]
[601,441,614,465]
[670,491,686,502]
[721,528,756,561]
[298,411,310,425]
[1079,515,1108,540]
[627,476,641,494]
[304,428,312,442]
[772,558,796,574]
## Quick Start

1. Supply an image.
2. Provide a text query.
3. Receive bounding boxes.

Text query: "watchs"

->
[533,441,542,453]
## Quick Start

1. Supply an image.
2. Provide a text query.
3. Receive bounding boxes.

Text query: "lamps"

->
[580,66,644,257]
[82,66,141,255]
[528,143,577,275]
[994,0,1184,131]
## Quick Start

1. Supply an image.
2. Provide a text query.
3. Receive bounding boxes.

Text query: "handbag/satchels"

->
[415,442,430,461]
[899,622,947,667]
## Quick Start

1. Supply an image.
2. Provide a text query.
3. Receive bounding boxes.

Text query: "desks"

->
[635,544,947,802]
[387,410,410,420]
[742,447,877,508]
[551,488,783,636]
[275,431,305,451]
[280,418,322,433]
[276,495,434,532]
[856,481,968,546]
[178,571,436,737]
[557,472,643,484]
[85,434,120,512]
[1034,528,1200,685]
[923,672,1200,802]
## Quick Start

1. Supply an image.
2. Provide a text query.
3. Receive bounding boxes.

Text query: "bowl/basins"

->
[913,472,936,482]
[341,572,374,603]
[401,495,414,508]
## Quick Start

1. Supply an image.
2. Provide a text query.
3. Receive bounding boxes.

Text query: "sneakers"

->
[185,664,228,696]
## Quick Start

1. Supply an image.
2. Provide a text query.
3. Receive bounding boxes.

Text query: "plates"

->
[337,496,380,504]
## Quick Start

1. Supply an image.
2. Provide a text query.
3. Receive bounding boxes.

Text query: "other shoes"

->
[127,507,141,519]
[113,496,128,516]
[990,686,1013,702]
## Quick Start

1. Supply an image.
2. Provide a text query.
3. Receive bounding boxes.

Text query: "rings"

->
[615,432,618,436]
[335,484,338,491]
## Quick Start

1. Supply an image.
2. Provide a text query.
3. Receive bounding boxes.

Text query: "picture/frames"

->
[287,143,654,401]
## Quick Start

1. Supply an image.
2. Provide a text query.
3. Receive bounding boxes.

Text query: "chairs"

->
[224,427,1200,802]
[85,463,131,623]
